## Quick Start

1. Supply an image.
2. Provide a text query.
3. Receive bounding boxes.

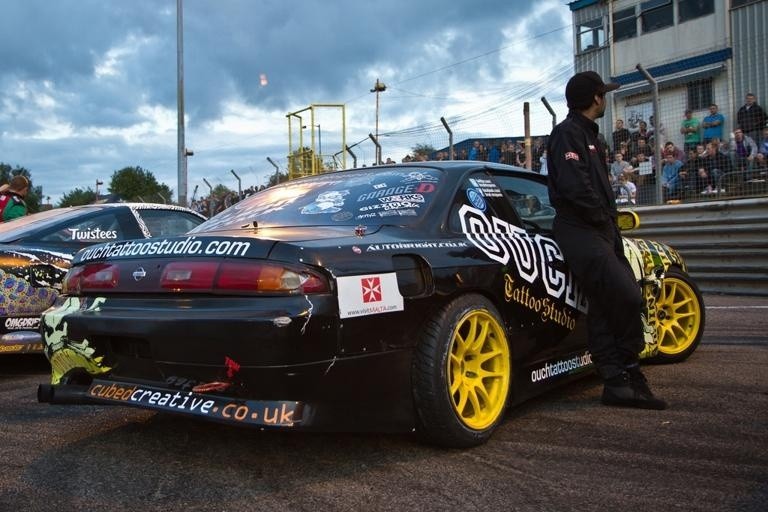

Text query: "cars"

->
[1,203,209,355]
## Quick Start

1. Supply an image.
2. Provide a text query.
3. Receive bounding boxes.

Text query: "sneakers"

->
[700,185,728,196]
[599,364,668,411]
[744,177,767,184]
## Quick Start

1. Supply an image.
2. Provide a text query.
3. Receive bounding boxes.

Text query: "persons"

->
[191,185,259,217]
[0,176,29,223]
[546,71,668,410]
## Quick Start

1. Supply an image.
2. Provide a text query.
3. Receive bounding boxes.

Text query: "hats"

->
[564,69,621,106]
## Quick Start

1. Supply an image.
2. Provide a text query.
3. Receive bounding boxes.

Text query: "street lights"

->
[94,176,103,205]
[301,123,322,162]
[370,78,391,165]
[36,156,705,452]
[184,147,195,206]
